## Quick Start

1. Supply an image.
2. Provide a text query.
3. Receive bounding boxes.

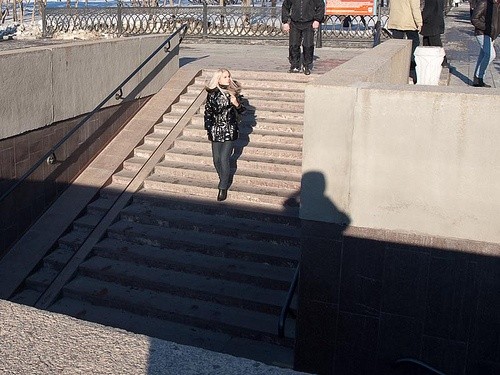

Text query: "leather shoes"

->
[217,188,227,200]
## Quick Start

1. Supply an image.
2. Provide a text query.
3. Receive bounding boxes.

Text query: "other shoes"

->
[287,67,301,72]
[302,65,311,75]
[473,76,491,87]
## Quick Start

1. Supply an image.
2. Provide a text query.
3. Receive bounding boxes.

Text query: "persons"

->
[281,0,325,75]
[386,0,424,73]
[470,0,500,87]
[420,0,449,68]
[204,67,247,202]
